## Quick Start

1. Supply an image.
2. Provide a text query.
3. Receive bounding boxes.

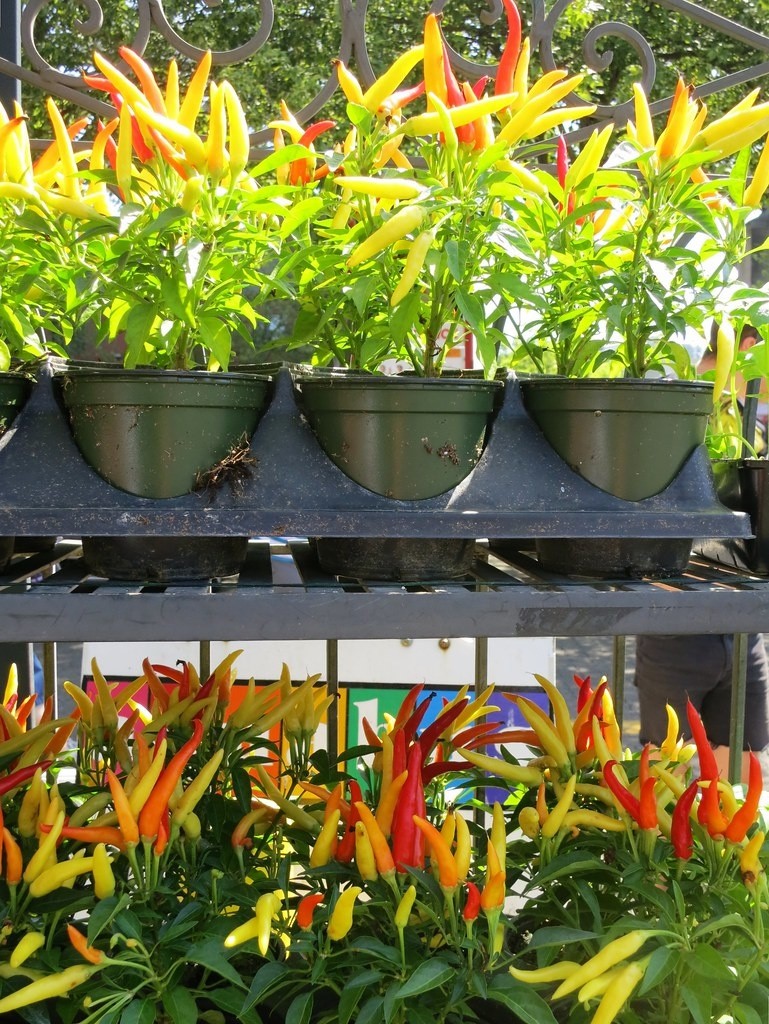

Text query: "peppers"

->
[1,0,767,1022]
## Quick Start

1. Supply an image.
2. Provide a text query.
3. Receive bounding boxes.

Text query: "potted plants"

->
[48,43,307,497]
[310,10,506,498]
[521,74,769,498]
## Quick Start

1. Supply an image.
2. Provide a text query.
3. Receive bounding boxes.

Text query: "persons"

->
[632,633,768,791]
[698,320,768,458]
